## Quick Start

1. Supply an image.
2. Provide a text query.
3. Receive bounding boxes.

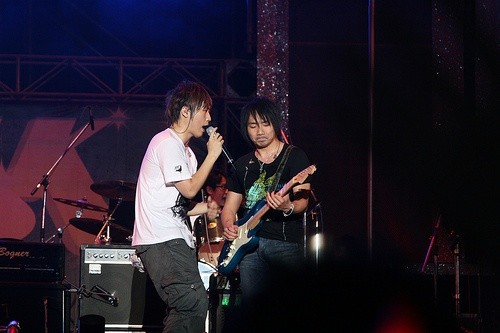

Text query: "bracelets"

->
[283,202,295,218]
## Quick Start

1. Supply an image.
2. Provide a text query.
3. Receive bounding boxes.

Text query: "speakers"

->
[0,279,72,333]
[79,243,147,331]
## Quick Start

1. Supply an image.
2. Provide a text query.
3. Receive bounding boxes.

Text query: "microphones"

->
[88,105,96,130]
[94,285,117,305]
[206,126,237,171]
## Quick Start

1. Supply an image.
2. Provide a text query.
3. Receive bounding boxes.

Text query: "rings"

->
[271,201,275,205]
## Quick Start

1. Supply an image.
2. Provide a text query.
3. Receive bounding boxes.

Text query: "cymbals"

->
[69,218,137,242]
[90,180,137,202]
[54,198,109,211]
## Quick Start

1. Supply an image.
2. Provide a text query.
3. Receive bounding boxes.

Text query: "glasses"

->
[215,184,228,191]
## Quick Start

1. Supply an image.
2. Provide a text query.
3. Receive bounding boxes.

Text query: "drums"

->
[194,212,225,268]
[197,260,219,333]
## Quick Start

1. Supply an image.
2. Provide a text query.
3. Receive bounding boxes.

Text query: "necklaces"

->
[255,142,280,175]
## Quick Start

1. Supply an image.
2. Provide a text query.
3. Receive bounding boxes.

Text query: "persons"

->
[132,81,224,333]
[221,99,312,300]
[191,169,239,243]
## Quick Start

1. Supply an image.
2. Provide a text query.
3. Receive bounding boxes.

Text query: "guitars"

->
[217,164,317,275]
